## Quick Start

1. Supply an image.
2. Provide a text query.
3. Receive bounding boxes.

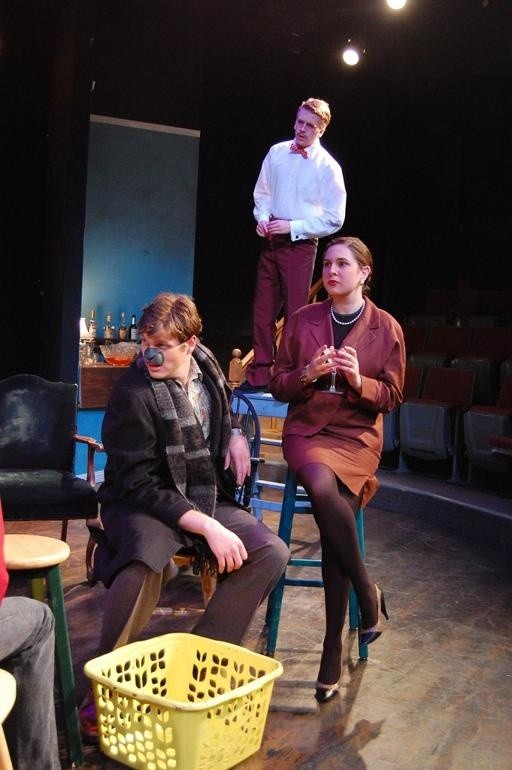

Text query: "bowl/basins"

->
[99,344,137,367]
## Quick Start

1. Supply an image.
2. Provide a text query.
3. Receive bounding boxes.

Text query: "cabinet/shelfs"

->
[77,337,147,411]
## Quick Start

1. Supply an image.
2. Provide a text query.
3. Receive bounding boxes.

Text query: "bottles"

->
[103,312,114,340]
[88,309,98,343]
[119,312,129,340]
[128,315,138,341]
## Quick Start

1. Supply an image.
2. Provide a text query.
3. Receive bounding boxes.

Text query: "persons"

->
[235,96,346,396]
[266,234,408,703]
[1,503,62,770]
[77,290,292,745]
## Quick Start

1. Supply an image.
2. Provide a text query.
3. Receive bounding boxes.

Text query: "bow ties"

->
[289,143,308,160]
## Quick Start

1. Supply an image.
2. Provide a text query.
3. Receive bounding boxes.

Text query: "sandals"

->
[313,643,344,704]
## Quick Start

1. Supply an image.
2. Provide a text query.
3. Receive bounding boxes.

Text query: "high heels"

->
[360,582,390,645]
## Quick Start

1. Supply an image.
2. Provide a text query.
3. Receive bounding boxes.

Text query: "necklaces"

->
[330,299,365,325]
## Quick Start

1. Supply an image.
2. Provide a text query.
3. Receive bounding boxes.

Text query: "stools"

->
[1,530,99,764]
[0,667,26,770]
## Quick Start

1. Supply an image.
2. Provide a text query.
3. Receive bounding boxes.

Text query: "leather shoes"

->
[78,687,100,747]
[234,379,270,395]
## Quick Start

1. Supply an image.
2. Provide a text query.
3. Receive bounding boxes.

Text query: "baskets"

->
[82,631,285,770]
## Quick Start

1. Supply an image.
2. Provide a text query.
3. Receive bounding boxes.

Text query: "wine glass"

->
[319,348,345,395]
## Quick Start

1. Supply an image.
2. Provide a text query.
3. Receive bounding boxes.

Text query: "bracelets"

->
[231,428,246,437]
[300,365,316,386]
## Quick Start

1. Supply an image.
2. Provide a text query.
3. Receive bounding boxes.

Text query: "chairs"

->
[1,372,106,585]
[379,352,512,501]
